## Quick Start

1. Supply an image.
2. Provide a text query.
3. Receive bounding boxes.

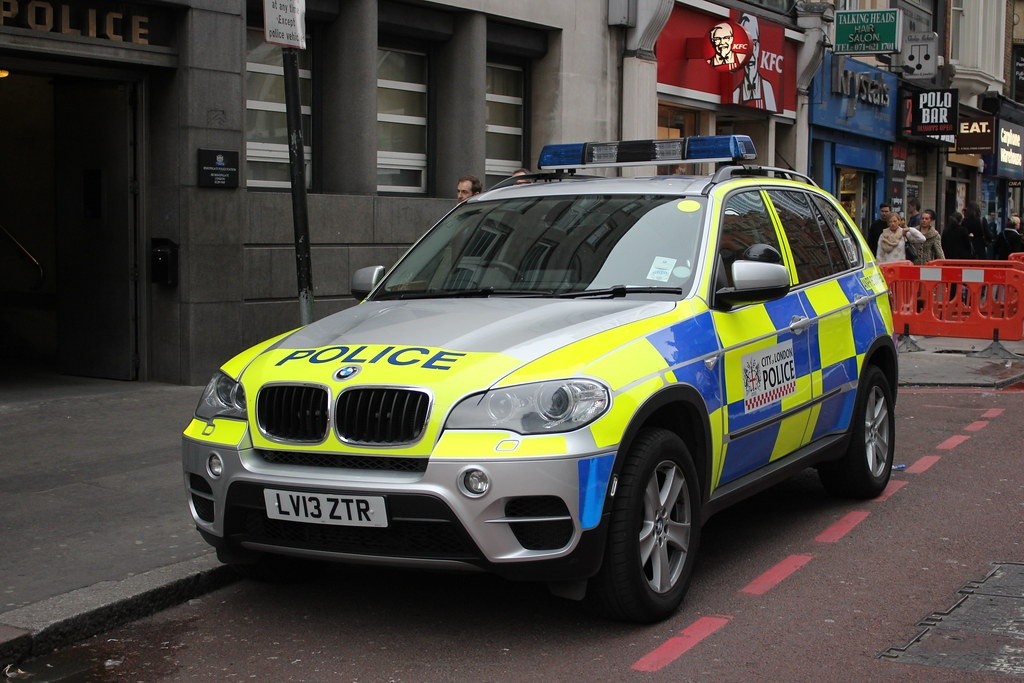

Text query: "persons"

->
[996,217,1024,260]
[867,204,892,256]
[876,212,926,263]
[940,211,974,259]
[906,198,1001,264]
[457,176,483,205]
[907,209,945,263]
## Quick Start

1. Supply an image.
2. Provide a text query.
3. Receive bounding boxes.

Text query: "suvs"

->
[180,135,899,624]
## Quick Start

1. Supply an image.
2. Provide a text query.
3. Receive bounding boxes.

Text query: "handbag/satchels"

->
[905,234,918,260]
[979,216,996,247]
